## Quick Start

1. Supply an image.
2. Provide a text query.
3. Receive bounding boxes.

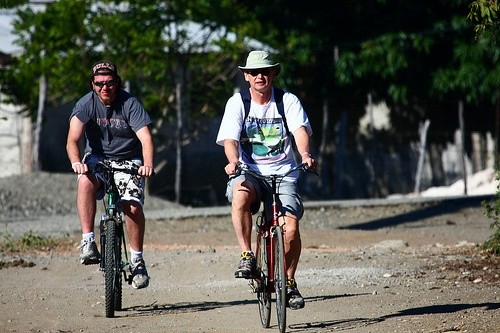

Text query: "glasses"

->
[246,69,273,76]
[93,79,116,87]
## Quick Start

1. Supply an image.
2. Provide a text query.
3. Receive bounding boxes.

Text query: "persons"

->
[66,61,154,288]
[216,51,315,309]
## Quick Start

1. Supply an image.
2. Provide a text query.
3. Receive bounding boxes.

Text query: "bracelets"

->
[72,162,81,168]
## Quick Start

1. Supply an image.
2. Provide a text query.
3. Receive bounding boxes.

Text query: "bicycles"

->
[225,163,322,333]
[72,162,157,319]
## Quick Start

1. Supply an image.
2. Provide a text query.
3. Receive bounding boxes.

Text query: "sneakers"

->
[234,252,255,278]
[78,237,100,265]
[129,258,149,289]
[286,279,305,310]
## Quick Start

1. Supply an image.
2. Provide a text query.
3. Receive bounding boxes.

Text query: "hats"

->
[238,51,281,77]
[92,61,117,77]
